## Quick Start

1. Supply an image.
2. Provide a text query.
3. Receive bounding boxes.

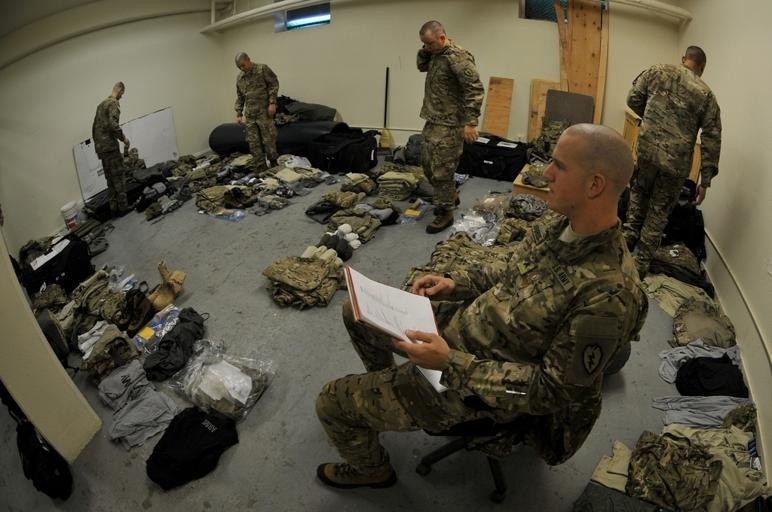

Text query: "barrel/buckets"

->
[60,200,79,231]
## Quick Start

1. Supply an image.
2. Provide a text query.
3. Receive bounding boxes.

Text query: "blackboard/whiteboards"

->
[73,107,180,204]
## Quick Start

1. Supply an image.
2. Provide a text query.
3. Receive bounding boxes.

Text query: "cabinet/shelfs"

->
[511,161,551,204]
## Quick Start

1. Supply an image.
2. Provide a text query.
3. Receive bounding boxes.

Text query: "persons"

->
[622,44,724,277]
[412,19,485,235]
[232,51,282,172]
[122,136,148,180]
[308,122,653,490]
[91,81,139,214]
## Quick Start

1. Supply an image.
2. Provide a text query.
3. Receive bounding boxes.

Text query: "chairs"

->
[414,285,650,503]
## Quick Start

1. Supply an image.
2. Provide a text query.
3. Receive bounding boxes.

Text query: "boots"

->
[126,291,154,336]
[434,199,460,217]
[224,191,245,210]
[426,210,455,234]
[232,188,253,207]
[147,261,172,303]
[151,271,187,311]
[315,456,398,492]
[118,288,141,332]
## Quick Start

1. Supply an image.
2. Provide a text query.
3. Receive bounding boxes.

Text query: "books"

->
[341,265,440,346]
